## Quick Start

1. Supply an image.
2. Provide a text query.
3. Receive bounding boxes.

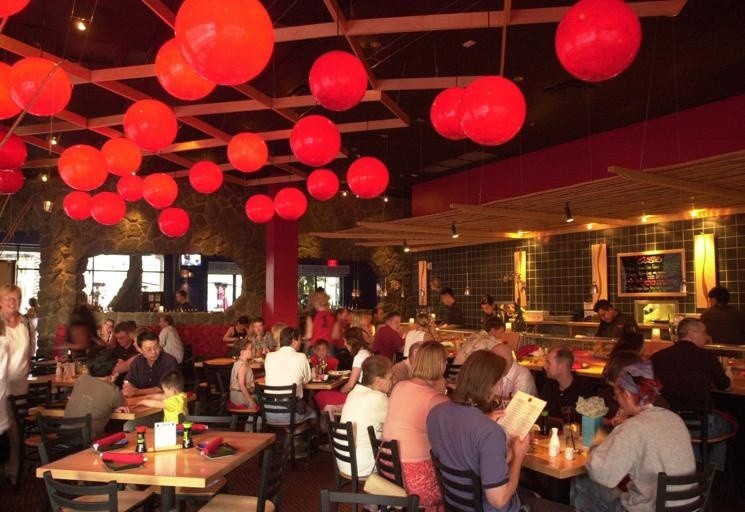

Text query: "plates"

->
[104,460,144,471]
[88,441,129,452]
[177,429,204,436]
[328,370,351,379]
[195,442,237,458]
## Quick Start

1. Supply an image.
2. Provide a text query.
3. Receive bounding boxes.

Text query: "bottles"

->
[540,411,549,436]
[55,361,62,376]
[78,360,89,375]
[135,427,147,453]
[67,348,73,363]
[307,355,313,376]
[182,421,193,449]
[548,427,560,458]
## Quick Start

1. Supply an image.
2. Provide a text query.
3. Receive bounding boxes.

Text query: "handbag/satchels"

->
[287,399,307,414]
[363,473,409,510]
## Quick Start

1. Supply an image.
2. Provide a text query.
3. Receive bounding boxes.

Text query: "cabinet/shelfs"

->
[526,320,678,342]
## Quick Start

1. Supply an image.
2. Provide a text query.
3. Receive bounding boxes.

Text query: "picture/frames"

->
[617,249,687,297]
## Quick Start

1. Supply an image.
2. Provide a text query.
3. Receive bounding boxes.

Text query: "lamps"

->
[401,254,405,298]
[591,230,598,293]
[459,0,527,145]
[451,223,459,239]
[384,270,388,297]
[555,1,642,82]
[350,256,360,299]
[403,239,410,252]
[464,246,471,296]
[431,29,467,142]
[564,201,574,223]
[521,241,527,296]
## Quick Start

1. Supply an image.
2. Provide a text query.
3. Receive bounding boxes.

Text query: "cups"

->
[651,328,661,341]
[718,355,728,373]
[562,423,580,446]
[565,448,577,460]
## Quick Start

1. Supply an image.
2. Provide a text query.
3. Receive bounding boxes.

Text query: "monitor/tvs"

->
[180,253,203,270]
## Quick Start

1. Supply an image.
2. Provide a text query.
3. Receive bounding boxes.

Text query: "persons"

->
[222,285,744,511]
[0,283,196,497]
